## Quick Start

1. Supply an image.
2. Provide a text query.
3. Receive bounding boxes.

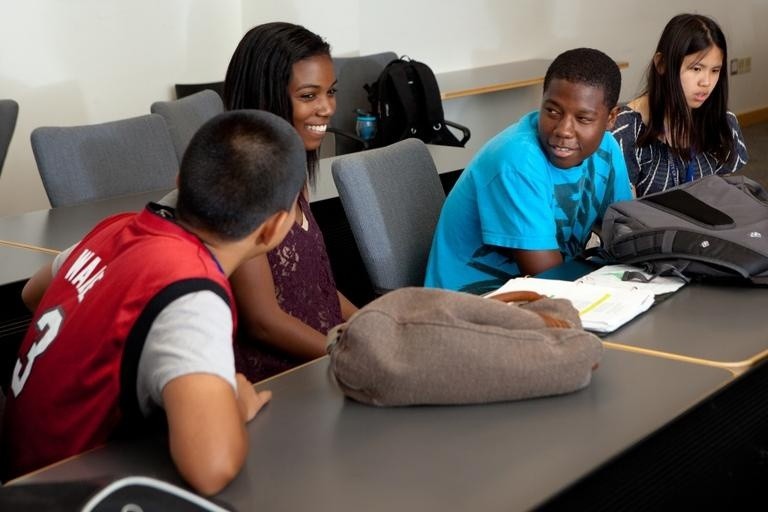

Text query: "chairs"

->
[318,51,398,158]
[30,88,227,208]
[333,132,445,300]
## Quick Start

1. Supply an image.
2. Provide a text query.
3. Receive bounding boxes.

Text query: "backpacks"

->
[600,173,768,286]
[362,55,465,148]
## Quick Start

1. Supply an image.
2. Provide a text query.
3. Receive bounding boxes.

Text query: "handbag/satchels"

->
[323,286,607,408]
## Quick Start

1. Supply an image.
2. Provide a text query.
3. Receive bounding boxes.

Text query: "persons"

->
[2,109,309,499]
[422,47,636,298]
[609,13,749,199]
[223,21,360,384]
